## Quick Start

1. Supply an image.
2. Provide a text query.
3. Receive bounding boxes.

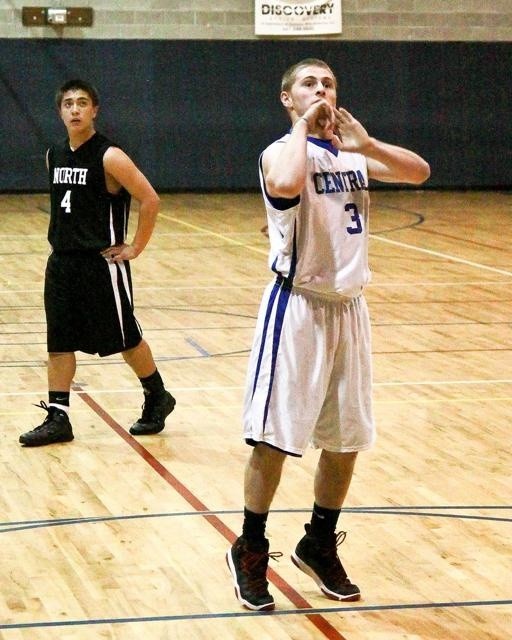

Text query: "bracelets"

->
[291,118,309,130]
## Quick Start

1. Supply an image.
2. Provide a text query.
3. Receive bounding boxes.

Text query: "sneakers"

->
[19,405,75,445]
[130,390,175,434]
[292,523,361,602]
[225,537,277,611]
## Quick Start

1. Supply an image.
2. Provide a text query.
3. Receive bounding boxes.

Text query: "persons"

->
[224,57,430,613]
[19,80,176,446]
